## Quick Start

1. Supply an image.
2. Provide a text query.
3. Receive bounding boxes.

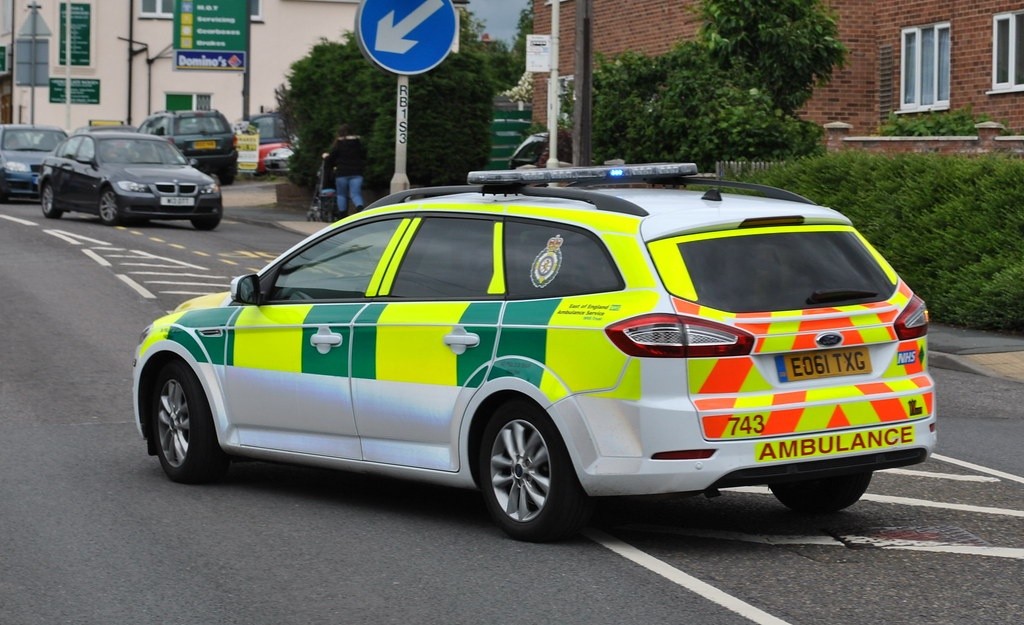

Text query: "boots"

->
[338,210,348,220]
[357,205,364,212]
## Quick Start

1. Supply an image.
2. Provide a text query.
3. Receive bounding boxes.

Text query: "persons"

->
[322,124,365,217]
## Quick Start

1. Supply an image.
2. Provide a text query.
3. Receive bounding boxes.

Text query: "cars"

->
[39,131,224,231]
[70,126,139,133]
[0,124,70,204]
[247,114,295,176]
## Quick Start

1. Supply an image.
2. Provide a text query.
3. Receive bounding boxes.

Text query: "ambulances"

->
[130,162,940,541]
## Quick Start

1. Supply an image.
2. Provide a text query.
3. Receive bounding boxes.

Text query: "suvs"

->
[137,110,237,186]
